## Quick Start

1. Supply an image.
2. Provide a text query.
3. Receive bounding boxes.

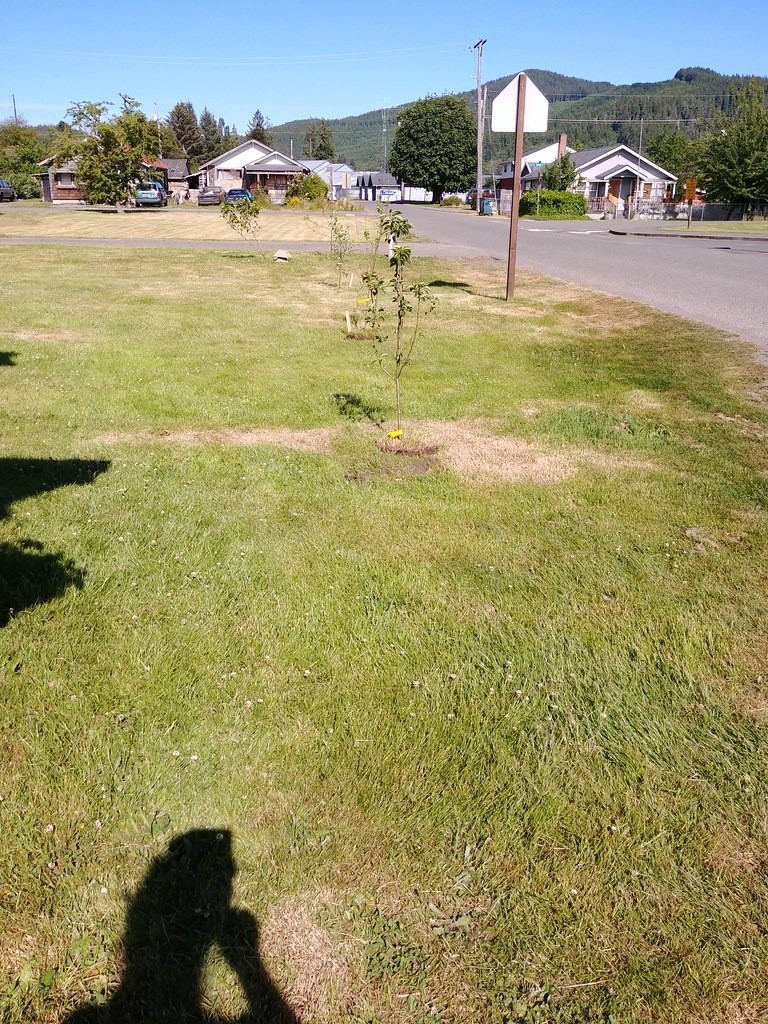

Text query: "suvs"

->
[465,188,494,210]
[0,179,16,203]
[134,181,168,208]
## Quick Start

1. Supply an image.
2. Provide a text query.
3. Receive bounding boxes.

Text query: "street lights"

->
[470,73,488,213]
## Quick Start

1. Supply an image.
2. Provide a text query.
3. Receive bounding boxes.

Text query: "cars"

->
[197,186,226,205]
[223,189,252,207]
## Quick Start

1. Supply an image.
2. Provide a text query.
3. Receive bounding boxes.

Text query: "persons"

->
[439,194,443,206]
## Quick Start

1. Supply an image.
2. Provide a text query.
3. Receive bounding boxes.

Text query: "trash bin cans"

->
[479,198,493,216]
